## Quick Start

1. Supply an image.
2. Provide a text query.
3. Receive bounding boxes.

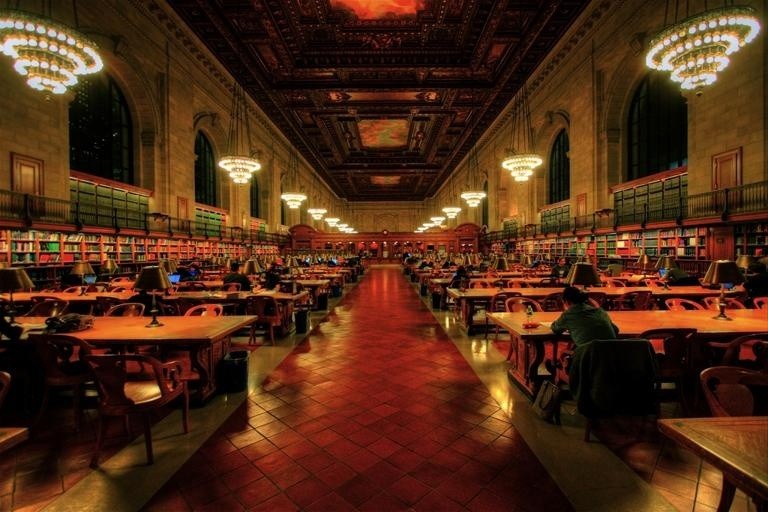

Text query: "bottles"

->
[527,304,534,323]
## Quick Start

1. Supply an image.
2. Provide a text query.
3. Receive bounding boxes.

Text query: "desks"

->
[446,286,746,337]
[404,254,630,292]
[1,316,258,404]
[430,278,656,311]
[132,255,363,311]
[658,418,768,512]
[0,290,309,347]
[485,309,768,402]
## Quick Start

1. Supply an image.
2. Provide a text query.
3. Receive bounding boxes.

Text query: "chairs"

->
[27,334,119,469]
[466,279,492,288]
[486,292,523,342]
[184,303,222,392]
[507,280,531,288]
[640,328,700,417]
[705,333,768,389]
[616,290,659,312]
[87,357,189,466]
[540,278,560,287]
[104,303,145,374]
[700,366,768,511]
[506,296,544,363]
[22,295,69,318]
[704,297,746,310]
[221,283,242,291]
[664,299,705,310]
[576,339,656,443]
[546,291,609,313]
[186,282,208,291]
[245,296,285,346]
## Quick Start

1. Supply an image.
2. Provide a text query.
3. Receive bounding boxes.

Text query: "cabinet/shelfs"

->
[0,220,281,291]
[516,209,768,278]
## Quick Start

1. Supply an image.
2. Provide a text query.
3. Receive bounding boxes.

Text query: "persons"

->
[551,257,568,278]
[264,267,280,290]
[551,287,618,386]
[661,261,690,285]
[327,257,335,267]
[222,262,251,291]
[450,267,470,288]
[418,257,433,270]
[480,257,490,272]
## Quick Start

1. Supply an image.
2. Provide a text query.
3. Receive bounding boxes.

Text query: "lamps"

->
[654,256,677,270]
[307,208,327,220]
[644,7,762,96]
[442,207,461,218]
[0,9,104,104]
[703,260,744,283]
[136,267,172,289]
[637,253,651,264]
[0,267,34,292]
[566,262,599,285]
[431,217,446,225]
[735,254,754,268]
[325,218,340,227]
[218,156,262,183]
[70,261,95,274]
[281,192,306,208]
[503,154,543,181]
[461,192,486,208]
[103,260,120,270]
